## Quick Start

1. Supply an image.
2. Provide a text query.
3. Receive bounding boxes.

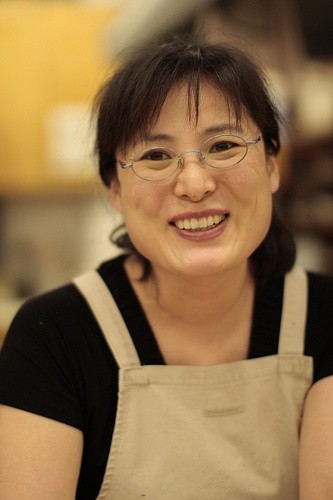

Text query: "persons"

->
[0,35,333,500]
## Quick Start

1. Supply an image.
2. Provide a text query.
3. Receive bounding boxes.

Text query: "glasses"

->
[115,134,265,181]
